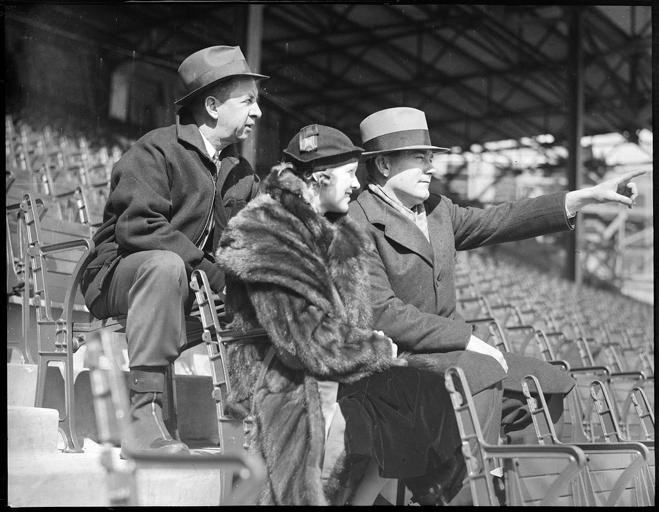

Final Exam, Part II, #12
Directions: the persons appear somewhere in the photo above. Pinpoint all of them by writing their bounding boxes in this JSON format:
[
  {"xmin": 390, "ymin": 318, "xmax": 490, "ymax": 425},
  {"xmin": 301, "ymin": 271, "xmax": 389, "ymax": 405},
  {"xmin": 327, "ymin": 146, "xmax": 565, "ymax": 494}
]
[
  {"xmin": 214, "ymin": 124, "xmax": 467, "ymax": 506},
  {"xmin": 79, "ymin": 45, "xmax": 270, "ymax": 460},
  {"xmin": 347, "ymin": 107, "xmax": 646, "ymax": 474}
]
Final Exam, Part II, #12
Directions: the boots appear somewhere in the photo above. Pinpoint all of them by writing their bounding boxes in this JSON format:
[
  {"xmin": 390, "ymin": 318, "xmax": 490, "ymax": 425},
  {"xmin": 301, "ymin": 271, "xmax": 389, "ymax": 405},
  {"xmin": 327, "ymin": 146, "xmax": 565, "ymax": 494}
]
[{"xmin": 119, "ymin": 366, "xmax": 190, "ymax": 461}]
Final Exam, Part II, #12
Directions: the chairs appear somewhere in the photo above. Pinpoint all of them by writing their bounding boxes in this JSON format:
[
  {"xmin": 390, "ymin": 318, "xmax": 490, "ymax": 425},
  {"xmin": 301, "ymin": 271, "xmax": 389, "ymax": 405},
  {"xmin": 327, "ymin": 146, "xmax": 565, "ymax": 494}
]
[{"xmin": 5, "ymin": 113, "xmax": 654, "ymax": 506}]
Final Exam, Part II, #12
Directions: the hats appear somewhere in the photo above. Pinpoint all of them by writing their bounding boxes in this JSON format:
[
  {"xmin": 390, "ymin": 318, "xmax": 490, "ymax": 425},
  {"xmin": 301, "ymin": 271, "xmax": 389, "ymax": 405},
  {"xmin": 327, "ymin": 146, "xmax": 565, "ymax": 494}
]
[
  {"xmin": 174, "ymin": 45, "xmax": 269, "ymax": 104},
  {"xmin": 361, "ymin": 106, "xmax": 451, "ymax": 154},
  {"xmin": 282, "ymin": 124, "xmax": 365, "ymax": 172}
]
